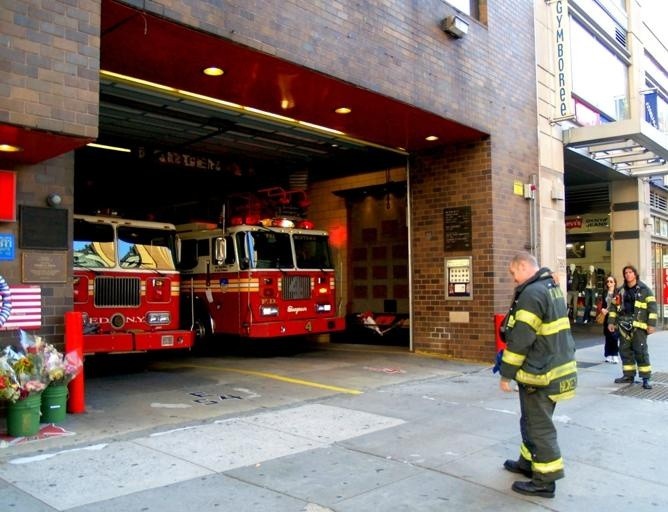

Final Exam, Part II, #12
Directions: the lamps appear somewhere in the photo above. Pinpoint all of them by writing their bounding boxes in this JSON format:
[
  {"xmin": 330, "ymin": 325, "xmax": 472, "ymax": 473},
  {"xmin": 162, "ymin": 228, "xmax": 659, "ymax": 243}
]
[{"xmin": 47, "ymin": 191, "xmax": 63, "ymax": 207}]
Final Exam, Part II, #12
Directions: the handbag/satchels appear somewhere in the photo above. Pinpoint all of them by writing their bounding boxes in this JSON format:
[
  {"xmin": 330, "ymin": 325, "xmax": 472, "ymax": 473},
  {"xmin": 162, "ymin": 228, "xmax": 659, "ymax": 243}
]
[{"xmin": 596, "ymin": 312, "xmax": 605, "ymax": 325}]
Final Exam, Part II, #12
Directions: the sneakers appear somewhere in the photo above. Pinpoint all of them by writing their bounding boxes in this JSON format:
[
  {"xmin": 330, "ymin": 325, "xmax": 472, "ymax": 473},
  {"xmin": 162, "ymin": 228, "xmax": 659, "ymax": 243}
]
[
  {"xmin": 612, "ymin": 356, "xmax": 618, "ymax": 364},
  {"xmin": 605, "ymin": 356, "xmax": 612, "ymax": 362}
]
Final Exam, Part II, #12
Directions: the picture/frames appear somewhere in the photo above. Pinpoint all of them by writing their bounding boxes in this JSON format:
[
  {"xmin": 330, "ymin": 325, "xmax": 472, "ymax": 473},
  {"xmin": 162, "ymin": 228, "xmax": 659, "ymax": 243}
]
[
  {"xmin": 18, "ymin": 202, "xmax": 69, "ymax": 251},
  {"xmin": 22, "ymin": 251, "xmax": 68, "ymax": 284},
  {"xmin": 0, "ymin": 169, "xmax": 17, "ymax": 223}
]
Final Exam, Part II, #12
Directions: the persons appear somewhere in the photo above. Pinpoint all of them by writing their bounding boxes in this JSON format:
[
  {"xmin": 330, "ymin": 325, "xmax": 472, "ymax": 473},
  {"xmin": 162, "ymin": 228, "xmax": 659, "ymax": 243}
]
[
  {"xmin": 581, "ymin": 265, "xmax": 599, "ymax": 324},
  {"xmin": 498, "ymin": 250, "xmax": 578, "ymax": 498},
  {"xmin": 567, "ymin": 264, "xmax": 579, "ymax": 324},
  {"xmin": 607, "ymin": 265, "xmax": 658, "ymax": 389},
  {"xmin": 600, "ymin": 276, "xmax": 619, "ymax": 363}
]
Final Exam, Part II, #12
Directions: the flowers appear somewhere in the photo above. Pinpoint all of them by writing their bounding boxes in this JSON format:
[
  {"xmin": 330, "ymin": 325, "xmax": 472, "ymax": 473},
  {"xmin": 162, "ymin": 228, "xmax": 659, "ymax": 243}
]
[{"xmin": 0, "ymin": 329, "xmax": 84, "ymax": 404}]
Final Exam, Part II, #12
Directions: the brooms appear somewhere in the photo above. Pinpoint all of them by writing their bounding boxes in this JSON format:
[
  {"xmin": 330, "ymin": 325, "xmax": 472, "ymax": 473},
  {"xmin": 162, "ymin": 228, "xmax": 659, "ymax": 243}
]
[{"xmin": 596, "ymin": 288, "xmax": 625, "ymax": 324}]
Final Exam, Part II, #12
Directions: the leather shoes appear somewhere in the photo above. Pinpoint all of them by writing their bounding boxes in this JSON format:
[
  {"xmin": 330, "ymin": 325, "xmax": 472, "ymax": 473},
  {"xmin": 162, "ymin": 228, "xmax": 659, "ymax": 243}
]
[
  {"xmin": 615, "ymin": 376, "xmax": 634, "ymax": 383},
  {"xmin": 503, "ymin": 460, "xmax": 532, "ymax": 478},
  {"xmin": 512, "ymin": 482, "xmax": 556, "ymax": 497},
  {"xmin": 643, "ymin": 378, "xmax": 652, "ymax": 389}
]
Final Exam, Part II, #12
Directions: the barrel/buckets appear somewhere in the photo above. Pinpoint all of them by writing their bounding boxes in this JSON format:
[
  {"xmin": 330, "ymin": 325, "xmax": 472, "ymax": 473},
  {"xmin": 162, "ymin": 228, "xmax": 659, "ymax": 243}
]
[
  {"xmin": 39, "ymin": 384, "xmax": 70, "ymax": 423},
  {"xmin": 5, "ymin": 391, "xmax": 43, "ymax": 437}
]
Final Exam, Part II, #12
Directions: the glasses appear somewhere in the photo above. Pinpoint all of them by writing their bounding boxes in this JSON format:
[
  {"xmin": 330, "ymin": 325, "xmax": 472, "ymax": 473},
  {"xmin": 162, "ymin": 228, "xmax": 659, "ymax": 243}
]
[{"xmin": 606, "ymin": 280, "xmax": 612, "ymax": 283}]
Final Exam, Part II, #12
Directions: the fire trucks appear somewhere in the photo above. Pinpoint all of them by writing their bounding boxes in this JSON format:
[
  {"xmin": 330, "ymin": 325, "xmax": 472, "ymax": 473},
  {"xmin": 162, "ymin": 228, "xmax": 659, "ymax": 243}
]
[
  {"xmin": 173, "ymin": 204, "xmax": 346, "ymax": 349},
  {"xmin": 73, "ymin": 208, "xmax": 195, "ymax": 357}
]
